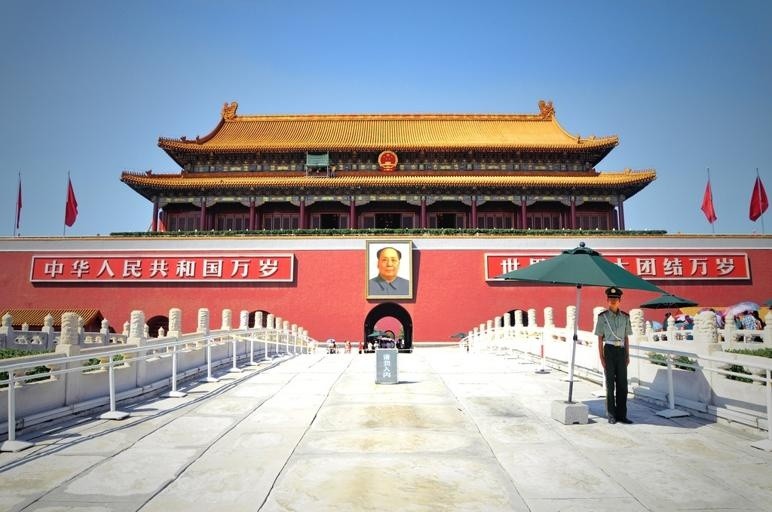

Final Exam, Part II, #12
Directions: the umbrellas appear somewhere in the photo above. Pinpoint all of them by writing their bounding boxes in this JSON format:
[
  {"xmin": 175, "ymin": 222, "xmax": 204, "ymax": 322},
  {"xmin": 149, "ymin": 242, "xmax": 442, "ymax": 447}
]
[
  {"xmin": 367, "ymin": 331, "xmax": 386, "ymax": 347},
  {"xmin": 448, "ymin": 332, "xmax": 470, "ymax": 338},
  {"xmin": 639, "ymin": 293, "xmax": 698, "ymax": 309}
]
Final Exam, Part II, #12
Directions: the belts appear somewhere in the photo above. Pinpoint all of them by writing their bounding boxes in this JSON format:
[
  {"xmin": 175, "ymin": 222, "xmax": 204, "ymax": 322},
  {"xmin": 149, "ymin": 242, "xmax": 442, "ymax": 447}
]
[{"xmin": 604, "ymin": 340, "xmax": 621, "ymax": 347}]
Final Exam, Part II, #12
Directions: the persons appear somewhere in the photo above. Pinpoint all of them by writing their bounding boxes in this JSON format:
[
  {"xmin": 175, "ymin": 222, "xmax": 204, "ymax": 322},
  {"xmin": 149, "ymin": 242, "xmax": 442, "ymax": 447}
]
[
  {"xmin": 594, "ymin": 286, "xmax": 635, "ymax": 425},
  {"xmin": 494, "ymin": 242, "xmax": 669, "ymax": 426},
  {"xmin": 323, "ymin": 338, "xmax": 404, "ymax": 354},
  {"xmin": 368, "ymin": 247, "xmax": 409, "ymax": 295},
  {"xmin": 663, "ymin": 299, "xmax": 772, "ymax": 340}
]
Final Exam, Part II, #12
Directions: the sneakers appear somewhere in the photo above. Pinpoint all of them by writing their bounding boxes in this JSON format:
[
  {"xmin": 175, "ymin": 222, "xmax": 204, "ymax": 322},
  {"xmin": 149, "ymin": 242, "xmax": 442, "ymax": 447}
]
[{"xmin": 608, "ymin": 415, "xmax": 633, "ymax": 424}]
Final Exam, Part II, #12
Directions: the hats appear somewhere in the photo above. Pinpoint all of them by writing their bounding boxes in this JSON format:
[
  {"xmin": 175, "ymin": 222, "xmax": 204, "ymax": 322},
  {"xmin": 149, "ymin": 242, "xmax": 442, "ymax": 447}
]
[{"xmin": 605, "ymin": 287, "xmax": 623, "ymax": 298}]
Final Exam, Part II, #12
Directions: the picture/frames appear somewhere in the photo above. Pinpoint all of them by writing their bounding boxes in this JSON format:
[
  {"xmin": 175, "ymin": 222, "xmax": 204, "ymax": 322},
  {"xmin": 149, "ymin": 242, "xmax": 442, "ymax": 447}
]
[{"xmin": 365, "ymin": 239, "xmax": 414, "ymax": 301}]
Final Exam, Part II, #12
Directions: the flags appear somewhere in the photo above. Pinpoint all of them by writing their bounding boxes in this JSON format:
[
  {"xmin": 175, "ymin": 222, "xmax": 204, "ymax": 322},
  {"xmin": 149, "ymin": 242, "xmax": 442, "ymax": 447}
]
[
  {"xmin": 64, "ymin": 179, "xmax": 79, "ymax": 227},
  {"xmin": 15, "ymin": 182, "xmax": 24, "ymax": 228},
  {"xmin": 750, "ymin": 176, "xmax": 769, "ymax": 222},
  {"xmin": 702, "ymin": 182, "xmax": 718, "ymax": 223}
]
